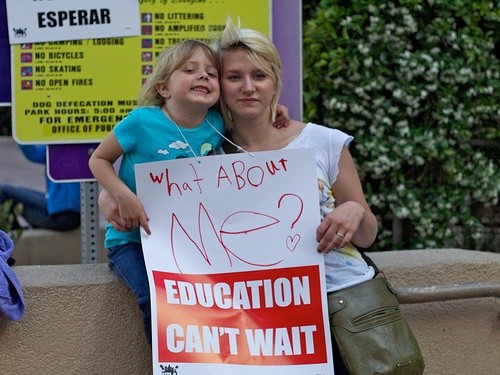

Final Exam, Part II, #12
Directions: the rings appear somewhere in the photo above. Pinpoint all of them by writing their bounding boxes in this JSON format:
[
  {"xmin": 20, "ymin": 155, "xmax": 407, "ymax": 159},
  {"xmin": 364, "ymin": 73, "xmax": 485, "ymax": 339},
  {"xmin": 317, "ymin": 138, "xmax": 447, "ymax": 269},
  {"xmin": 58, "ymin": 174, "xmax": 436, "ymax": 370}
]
[{"xmin": 337, "ymin": 232, "xmax": 345, "ymax": 239}]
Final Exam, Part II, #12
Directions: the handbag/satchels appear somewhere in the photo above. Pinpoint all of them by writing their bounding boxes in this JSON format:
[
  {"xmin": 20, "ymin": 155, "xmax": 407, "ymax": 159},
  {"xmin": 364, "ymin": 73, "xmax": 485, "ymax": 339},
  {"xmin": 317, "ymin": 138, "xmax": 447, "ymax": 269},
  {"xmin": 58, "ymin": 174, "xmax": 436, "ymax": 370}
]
[{"xmin": 328, "ymin": 272, "xmax": 424, "ymax": 374}]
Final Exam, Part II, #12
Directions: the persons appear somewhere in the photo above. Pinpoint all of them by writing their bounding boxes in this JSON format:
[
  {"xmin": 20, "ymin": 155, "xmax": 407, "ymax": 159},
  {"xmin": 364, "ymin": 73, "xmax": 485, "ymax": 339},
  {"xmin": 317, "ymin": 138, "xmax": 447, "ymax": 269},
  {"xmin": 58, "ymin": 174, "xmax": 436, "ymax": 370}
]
[
  {"xmin": 86, "ymin": 37, "xmax": 290, "ymax": 351},
  {"xmin": 96, "ymin": 16, "xmax": 423, "ymax": 374}
]
